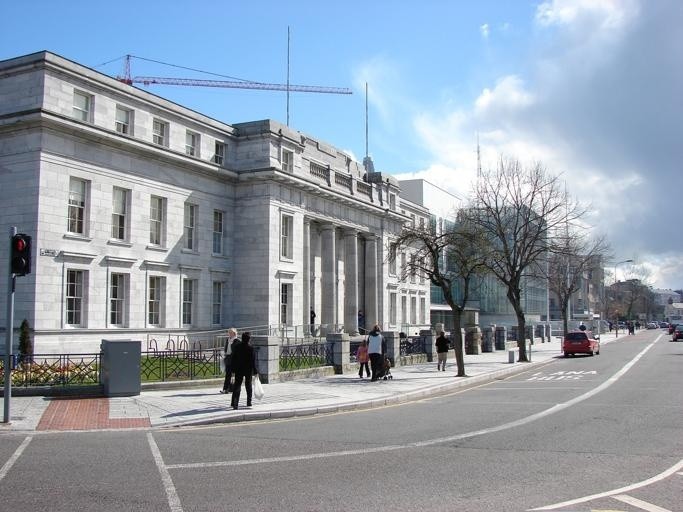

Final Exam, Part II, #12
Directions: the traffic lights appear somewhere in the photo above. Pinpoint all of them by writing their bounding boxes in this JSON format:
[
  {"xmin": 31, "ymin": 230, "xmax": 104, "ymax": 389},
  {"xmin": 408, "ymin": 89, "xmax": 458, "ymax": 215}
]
[{"xmin": 9, "ymin": 231, "xmax": 32, "ymax": 278}]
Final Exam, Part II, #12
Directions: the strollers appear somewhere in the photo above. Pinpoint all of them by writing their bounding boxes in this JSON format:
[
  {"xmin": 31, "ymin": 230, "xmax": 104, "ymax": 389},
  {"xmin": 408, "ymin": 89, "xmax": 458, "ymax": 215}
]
[{"xmin": 376, "ymin": 339, "xmax": 392, "ymax": 381}]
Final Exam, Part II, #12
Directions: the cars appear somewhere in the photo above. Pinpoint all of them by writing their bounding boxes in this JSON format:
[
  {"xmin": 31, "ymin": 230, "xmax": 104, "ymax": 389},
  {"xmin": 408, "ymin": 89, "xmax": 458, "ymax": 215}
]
[{"xmin": 606, "ymin": 315, "xmax": 682, "ymax": 342}]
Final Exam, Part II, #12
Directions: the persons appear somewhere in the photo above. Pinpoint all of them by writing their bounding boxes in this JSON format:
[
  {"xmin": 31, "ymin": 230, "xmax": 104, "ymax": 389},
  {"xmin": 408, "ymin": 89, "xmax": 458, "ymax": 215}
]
[
  {"xmin": 219, "ymin": 326, "xmax": 240, "ymax": 394},
  {"xmin": 366, "ymin": 324, "xmax": 387, "ymax": 382},
  {"xmin": 229, "ymin": 331, "xmax": 256, "ymax": 410},
  {"xmin": 357, "ymin": 310, "xmax": 364, "ymax": 332},
  {"xmin": 608, "ymin": 321, "xmax": 613, "ymax": 332},
  {"xmin": 356, "ymin": 339, "xmax": 370, "ymax": 378},
  {"xmin": 434, "ymin": 331, "xmax": 450, "ymax": 372},
  {"xmin": 628, "ymin": 321, "xmax": 641, "ymax": 335},
  {"xmin": 578, "ymin": 322, "xmax": 586, "ymax": 331},
  {"xmin": 310, "ymin": 306, "xmax": 316, "ymax": 333}
]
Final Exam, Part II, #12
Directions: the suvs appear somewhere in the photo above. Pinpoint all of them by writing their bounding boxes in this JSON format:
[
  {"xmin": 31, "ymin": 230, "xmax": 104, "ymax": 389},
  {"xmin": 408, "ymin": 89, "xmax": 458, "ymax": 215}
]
[{"xmin": 562, "ymin": 329, "xmax": 601, "ymax": 357}]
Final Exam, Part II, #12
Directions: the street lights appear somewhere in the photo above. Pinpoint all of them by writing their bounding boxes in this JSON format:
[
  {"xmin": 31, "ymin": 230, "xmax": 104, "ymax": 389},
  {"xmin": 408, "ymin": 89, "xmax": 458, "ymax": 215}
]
[{"xmin": 613, "ymin": 259, "xmax": 633, "ymax": 304}]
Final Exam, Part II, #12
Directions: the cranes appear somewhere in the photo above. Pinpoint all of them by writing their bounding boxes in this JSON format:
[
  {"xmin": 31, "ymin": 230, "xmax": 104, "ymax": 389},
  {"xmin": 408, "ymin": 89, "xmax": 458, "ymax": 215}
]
[{"xmin": 91, "ymin": 49, "xmax": 356, "ymax": 98}]
